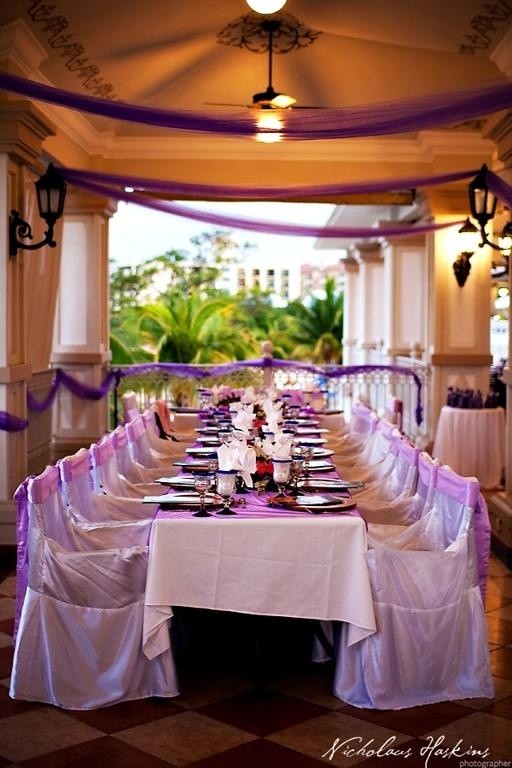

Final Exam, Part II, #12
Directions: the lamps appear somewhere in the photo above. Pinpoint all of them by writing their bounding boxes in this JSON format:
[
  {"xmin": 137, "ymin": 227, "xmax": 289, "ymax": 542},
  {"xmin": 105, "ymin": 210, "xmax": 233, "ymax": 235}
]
[
  {"xmin": 465, "ymin": 162, "xmax": 512, "ymax": 251},
  {"xmin": 452, "ymin": 216, "xmax": 482, "ymax": 287},
  {"xmin": 9, "ymin": 159, "xmax": 66, "ymax": 256}
]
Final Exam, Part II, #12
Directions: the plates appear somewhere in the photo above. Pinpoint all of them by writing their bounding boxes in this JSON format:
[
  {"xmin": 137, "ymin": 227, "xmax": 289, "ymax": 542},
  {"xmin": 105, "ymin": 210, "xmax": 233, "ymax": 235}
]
[
  {"xmin": 156, "ymin": 414, "xmax": 230, "ymax": 509},
  {"xmin": 277, "ymin": 416, "xmax": 366, "ymax": 511}
]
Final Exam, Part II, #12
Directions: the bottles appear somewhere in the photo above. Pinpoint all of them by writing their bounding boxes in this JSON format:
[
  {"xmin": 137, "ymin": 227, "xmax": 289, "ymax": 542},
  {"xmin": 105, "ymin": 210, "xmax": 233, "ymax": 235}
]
[{"xmin": 446, "ymin": 385, "xmax": 502, "ymax": 409}]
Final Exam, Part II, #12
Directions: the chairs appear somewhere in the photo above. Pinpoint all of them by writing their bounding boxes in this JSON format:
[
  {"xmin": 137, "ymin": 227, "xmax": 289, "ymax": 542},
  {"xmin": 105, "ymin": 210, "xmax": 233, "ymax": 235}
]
[{"xmin": 8, "ymin": 389, "xmax": 497, "ymax": 714}]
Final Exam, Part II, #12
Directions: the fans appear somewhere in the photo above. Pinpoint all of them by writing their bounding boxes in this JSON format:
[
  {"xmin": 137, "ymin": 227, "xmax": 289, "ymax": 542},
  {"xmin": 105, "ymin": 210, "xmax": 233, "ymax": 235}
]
[{"xmin": 200, "ymin": 8, "xmax": 332, "ymax": 114}]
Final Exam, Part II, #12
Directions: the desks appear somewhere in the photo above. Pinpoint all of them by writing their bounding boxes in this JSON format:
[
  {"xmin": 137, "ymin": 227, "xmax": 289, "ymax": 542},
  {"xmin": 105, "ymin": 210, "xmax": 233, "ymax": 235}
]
[{"xmin": 431, "ymin": 405, "xmax": 506, "ymax": 492}]
